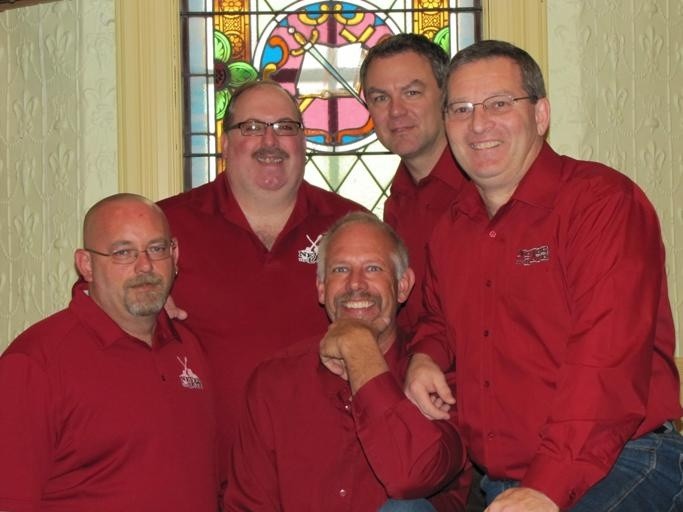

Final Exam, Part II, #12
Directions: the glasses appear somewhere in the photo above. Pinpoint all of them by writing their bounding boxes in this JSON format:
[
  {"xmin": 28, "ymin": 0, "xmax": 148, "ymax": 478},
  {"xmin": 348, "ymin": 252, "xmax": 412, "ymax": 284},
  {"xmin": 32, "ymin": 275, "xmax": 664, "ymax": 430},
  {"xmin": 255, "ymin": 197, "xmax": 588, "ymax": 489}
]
[
  {"xmin": 91, "ymin": 239, "xmax": 175, "ymax": 267},
  {"xmin": 225, "ymin": 119, "xmax": 302, "ymax": 138},
  {"xmin": 441, "ymin": 93, "xmax": 534, "ymax": 122}
]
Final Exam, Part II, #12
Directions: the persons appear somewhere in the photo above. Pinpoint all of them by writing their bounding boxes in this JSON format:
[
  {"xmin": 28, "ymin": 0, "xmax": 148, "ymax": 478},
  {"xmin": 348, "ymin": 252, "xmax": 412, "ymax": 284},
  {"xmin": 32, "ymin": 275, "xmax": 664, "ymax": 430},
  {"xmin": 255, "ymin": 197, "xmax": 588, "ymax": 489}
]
[
  {"xmin": 156, "ymin": 80, "xmax": 378, "ymax": 480},
  {"xmin": 0, "ymin": 193, "xmax": 219, "ymax": 512},
  {"xmin": 357, "ymin": 33, "xmax": 472, "ymax": 367},
  {"xmin": 399, "ymin": 38, "xmax": 682, "ymax": 512},
  {"xmin": 220, "ymin": 210, "xmax": 474, "ymax": 511}
]
